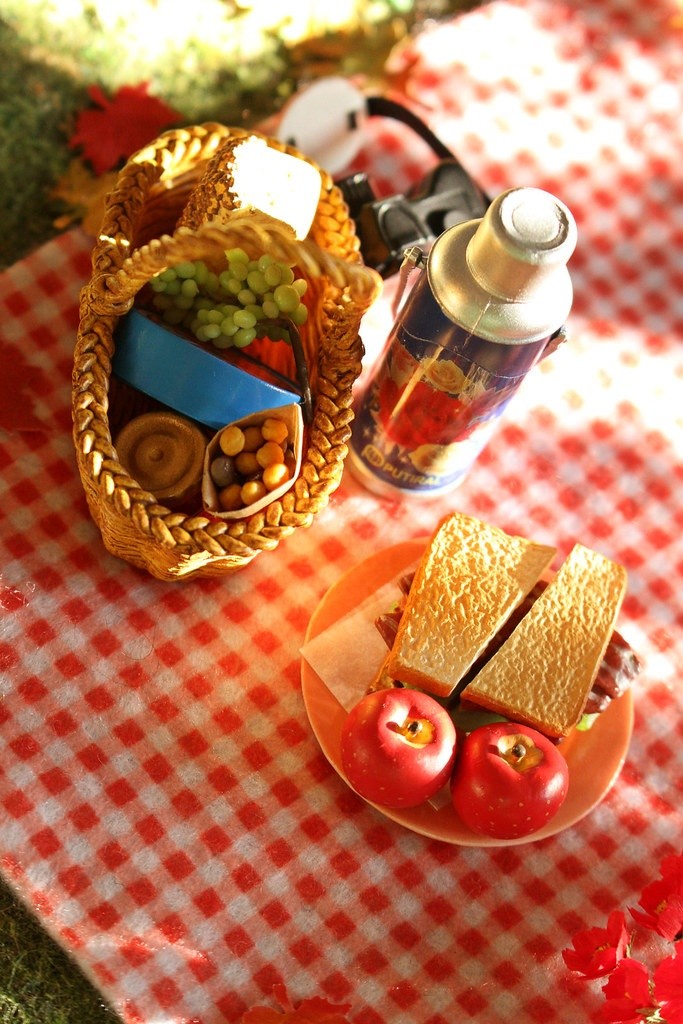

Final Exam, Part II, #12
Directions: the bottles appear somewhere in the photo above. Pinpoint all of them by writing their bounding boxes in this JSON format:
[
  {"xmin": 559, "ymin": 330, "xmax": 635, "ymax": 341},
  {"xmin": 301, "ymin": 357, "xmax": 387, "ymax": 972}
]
[{"xmin": 346, "ymin": 185, "xmax": 577, "ymax": 504}]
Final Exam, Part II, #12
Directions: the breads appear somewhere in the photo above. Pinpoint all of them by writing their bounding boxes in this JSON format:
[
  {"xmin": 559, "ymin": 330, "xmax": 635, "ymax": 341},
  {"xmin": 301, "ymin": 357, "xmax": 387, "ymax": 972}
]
[
  {"xmin": 176, "ymin": 136, "xmax": 323, "ymax": 242},
  {"xmin": 387, "ymin": 510, "xmax": 557, "ymax": 695},
  {"xmin": 460, "ymin": 544, "xmax": 627, "ymax": 738}
]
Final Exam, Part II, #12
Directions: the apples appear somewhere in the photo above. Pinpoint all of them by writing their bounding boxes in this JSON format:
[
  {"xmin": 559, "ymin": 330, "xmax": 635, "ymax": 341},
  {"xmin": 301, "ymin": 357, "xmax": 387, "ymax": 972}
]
[
  {"xmin": 338, "ymin": 689, "xmax": 460, "ymax": 809},
  {"xmin": 448, "ymin": 721, "xmax": 568, "ymax": 839}
]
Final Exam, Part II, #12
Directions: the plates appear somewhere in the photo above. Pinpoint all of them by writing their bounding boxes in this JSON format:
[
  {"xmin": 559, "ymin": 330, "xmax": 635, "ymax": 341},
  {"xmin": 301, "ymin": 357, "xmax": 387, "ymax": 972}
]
[{"xmin": 300, "ymin": 534, "xmax": 635, "ymax": 847}]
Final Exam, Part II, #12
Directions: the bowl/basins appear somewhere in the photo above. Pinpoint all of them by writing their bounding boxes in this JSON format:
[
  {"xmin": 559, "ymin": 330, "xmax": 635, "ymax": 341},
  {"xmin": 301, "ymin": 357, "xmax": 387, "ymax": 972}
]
[{"xmin": 114, "ymin": 305, "xmax": 305, "ymax": 433}]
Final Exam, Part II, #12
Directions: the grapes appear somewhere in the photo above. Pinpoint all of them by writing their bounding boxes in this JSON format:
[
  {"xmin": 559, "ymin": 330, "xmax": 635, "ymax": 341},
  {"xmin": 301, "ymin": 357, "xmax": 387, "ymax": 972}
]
[{"xmin": 146, "ymin": 247, "xmax": 308, "ymax": 348}]
